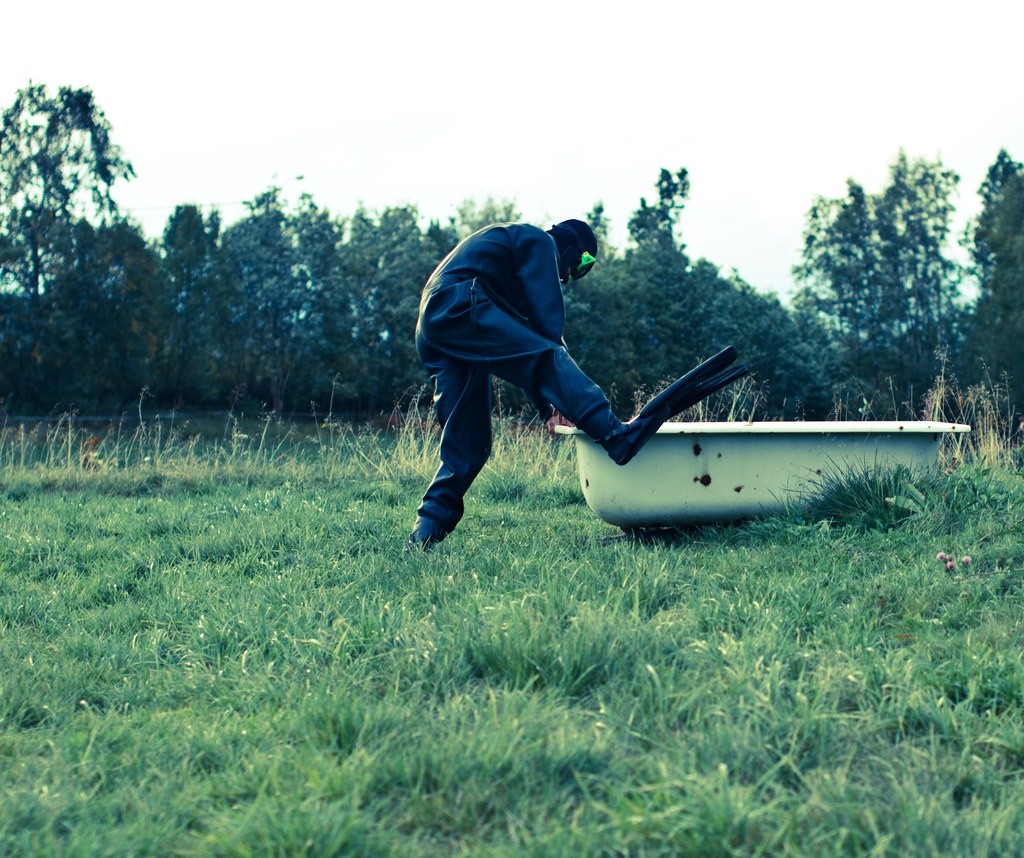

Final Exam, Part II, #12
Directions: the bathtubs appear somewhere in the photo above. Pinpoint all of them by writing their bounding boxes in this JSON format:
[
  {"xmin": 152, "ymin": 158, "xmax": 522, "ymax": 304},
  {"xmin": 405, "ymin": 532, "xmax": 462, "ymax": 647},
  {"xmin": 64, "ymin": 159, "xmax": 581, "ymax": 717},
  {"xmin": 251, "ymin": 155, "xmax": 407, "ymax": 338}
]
[{"xmin": 552, "ymin": 421, "xmax": 971, "ymax": 532}]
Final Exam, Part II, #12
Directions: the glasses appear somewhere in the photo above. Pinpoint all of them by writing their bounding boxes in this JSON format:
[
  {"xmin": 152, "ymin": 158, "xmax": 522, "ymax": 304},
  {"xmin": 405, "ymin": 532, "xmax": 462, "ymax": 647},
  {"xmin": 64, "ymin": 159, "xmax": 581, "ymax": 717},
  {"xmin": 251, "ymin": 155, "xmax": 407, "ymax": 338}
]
[{"xmin": 574, "ymin": 251, "xmax": 595, "ymax": 282}]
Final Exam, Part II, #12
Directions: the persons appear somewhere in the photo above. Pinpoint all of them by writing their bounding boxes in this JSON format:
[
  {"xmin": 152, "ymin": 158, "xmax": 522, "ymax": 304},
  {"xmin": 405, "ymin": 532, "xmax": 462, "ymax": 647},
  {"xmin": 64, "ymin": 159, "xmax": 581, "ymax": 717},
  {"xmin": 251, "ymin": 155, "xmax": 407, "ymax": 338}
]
[{"xmin": 404, "ymin": 219, "xmax": 751, "ymax": 556}]
